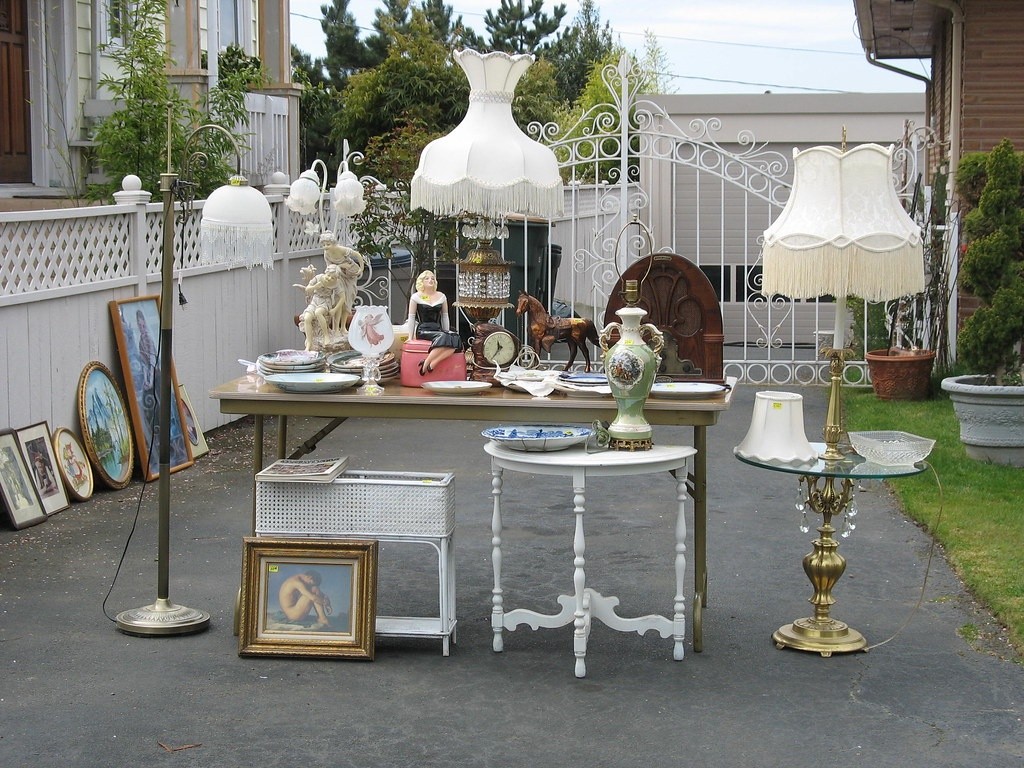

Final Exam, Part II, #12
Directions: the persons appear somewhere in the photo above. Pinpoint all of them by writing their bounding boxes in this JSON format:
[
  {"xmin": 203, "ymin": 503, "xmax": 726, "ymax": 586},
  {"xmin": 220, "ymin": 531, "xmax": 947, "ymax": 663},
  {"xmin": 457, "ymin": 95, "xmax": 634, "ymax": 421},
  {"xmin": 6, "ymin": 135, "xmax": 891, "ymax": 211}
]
[
  {"xmin": 404, "ymin": 271, "xmax": 461, "ymax": 375},
  {"xmin": 320, "ymin": 232, "xmax": 364, "ymax": 334},
  {"xmin": 303, "ymin": 264, "xmax": 346, "ymax": 352}
]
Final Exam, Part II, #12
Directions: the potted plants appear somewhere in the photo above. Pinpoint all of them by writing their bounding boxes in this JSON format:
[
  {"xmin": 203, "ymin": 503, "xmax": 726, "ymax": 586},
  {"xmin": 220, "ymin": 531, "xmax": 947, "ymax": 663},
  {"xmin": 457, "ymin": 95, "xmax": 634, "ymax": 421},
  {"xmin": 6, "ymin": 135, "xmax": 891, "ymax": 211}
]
[
  {"xmin": 941, "ymin": 139, "xmax": 1024, "ymax": 466},
  {"xmin": 866, "ymin": 160, "xmax": 947, "ymax": 399}
]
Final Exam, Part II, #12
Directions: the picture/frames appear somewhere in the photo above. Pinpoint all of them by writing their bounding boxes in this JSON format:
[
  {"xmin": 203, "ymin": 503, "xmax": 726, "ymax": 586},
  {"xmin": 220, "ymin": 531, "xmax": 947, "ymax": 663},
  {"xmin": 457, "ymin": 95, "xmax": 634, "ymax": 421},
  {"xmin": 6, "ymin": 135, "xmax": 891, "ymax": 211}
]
[
  {"xmin": 17, "ymin": 421, "xmax": 70, "ymax": 517},
  {"xmin": 0, "ymin": 427, "xmax": 48, "ymax": 531},
  {"xmin": 238, "ymin": 537, "xmax": 379, "ymax": 662},
  {"xmin": 108, "ymin": 294, "xmax": 195, "ymax": 482},
  {"xmin": 178, "ymin": 384, "xmax": 209, "ymax": 459}
]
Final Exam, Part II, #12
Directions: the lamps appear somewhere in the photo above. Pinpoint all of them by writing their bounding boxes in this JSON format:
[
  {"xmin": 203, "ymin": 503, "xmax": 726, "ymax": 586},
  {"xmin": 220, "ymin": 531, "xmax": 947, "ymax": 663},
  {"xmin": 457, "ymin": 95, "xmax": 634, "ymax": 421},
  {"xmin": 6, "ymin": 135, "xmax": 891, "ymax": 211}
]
[
  {"xmin": 762, "ymin": 127, "xmax": 924, "ymax": 460},
  {"xmin": 411, "ymin": 48, "xmax": 564, "ymax": 367},
  {"xmin": 283, "ymin": 139, "xmax": 365, "ymax": 356},
  {"xmin": 101, "ymin": 101, "xmax": 277, "ymax": 638}
]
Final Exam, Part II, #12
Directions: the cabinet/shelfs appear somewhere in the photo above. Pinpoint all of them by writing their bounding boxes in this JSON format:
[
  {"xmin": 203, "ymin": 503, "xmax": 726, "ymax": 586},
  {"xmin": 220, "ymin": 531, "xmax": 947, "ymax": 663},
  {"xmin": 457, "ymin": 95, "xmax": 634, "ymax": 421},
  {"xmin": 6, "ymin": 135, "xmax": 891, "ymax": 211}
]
[{"xmin": 258, "ymin": 472, "xmax": 459, "ymax": 657}]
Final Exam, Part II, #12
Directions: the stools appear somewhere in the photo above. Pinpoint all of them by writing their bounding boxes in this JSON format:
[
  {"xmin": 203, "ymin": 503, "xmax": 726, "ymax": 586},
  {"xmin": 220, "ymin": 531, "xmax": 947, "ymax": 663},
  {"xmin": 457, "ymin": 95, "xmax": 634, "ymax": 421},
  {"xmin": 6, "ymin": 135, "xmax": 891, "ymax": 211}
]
[{"xmin": 402, "ymin": 341, "xmax": 469, "ymax": 385}]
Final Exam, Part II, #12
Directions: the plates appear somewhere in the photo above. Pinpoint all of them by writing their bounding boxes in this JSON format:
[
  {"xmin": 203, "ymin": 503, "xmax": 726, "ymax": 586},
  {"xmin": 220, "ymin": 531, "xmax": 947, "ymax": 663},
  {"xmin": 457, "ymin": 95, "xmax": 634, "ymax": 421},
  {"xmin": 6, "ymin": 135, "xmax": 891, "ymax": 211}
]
[
  {"xmin": 257, "ymin": 349, "xmax": 398, "ymax": 393},
  {"xmin": 497, "ymin": 371, "xmax": 612, "ymax": 397},
  {"xmin": 482, "ymin": 427, "xmax": 595, "ymax": 452},
  {"xmin": 650, "ymin": 383, "xmax": 724, "ymax": 396},
  {"xmin": 421, "ymin": 380, "xmax": 492, "ymax": 395}
]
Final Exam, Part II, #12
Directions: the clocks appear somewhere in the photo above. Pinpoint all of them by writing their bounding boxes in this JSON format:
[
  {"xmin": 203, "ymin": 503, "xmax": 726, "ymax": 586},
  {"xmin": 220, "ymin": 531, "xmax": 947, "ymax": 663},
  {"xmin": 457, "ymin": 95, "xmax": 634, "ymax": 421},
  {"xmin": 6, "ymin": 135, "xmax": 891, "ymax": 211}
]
[{"xmin": 468, "ymin": 322, "xmax": 520, "ymax": 385}]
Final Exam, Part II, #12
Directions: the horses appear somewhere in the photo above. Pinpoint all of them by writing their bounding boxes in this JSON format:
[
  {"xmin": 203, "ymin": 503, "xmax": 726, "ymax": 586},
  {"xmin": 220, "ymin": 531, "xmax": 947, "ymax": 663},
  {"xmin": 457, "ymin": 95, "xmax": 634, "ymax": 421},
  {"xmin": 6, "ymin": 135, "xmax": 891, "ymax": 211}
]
[{"xmin": 515, "ymin": 289, "xmax": 601, "ymax": 372}]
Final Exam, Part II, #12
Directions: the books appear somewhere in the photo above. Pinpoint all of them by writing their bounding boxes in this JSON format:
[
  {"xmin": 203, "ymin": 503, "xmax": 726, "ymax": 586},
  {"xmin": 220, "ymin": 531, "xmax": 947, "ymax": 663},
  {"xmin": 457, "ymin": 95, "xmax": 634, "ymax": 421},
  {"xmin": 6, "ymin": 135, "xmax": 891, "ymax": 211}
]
[{"xmin": 255, "ymin": 457, "xmax": 349, "ymax": 484}]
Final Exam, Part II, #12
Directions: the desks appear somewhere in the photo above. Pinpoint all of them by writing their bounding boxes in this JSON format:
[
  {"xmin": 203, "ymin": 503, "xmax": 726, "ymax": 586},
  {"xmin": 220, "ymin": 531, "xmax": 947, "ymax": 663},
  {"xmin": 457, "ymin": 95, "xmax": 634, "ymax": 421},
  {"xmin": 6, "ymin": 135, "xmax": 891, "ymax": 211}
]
[
  {"xmin": 211, "ymin": 373, "xmax": 738, "ymax": 655},
  {"xmin": 485, "ymin": 441, "xmax": 698, "ymax": 678},
  {"xmin": 736, "ymin": 443, "xmax": 927, "ymax": 658}
]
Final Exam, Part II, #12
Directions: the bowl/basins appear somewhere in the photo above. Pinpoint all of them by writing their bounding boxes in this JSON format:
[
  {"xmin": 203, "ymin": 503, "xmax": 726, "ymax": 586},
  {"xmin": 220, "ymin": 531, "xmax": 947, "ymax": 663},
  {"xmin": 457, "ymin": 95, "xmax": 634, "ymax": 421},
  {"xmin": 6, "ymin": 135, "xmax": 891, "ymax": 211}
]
[{"xmin": 848, "ymin": 429, "xmax": 936, "ymax": 466}]
